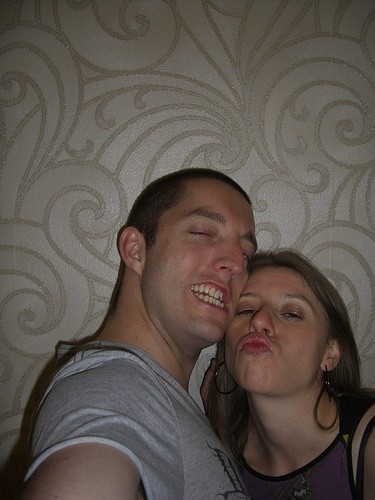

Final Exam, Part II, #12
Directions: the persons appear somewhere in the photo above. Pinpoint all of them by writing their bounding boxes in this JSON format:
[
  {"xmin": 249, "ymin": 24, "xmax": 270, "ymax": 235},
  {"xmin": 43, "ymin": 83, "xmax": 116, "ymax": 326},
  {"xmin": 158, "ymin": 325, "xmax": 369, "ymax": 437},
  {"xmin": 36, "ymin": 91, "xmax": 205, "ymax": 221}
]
[
  {"xmin": 206, "ymin": 250, "xmax": 375, "ymax": 500},
  {"xmin": 21, "ymin": 169, "xmax": 257, "ymax": 500}
]
